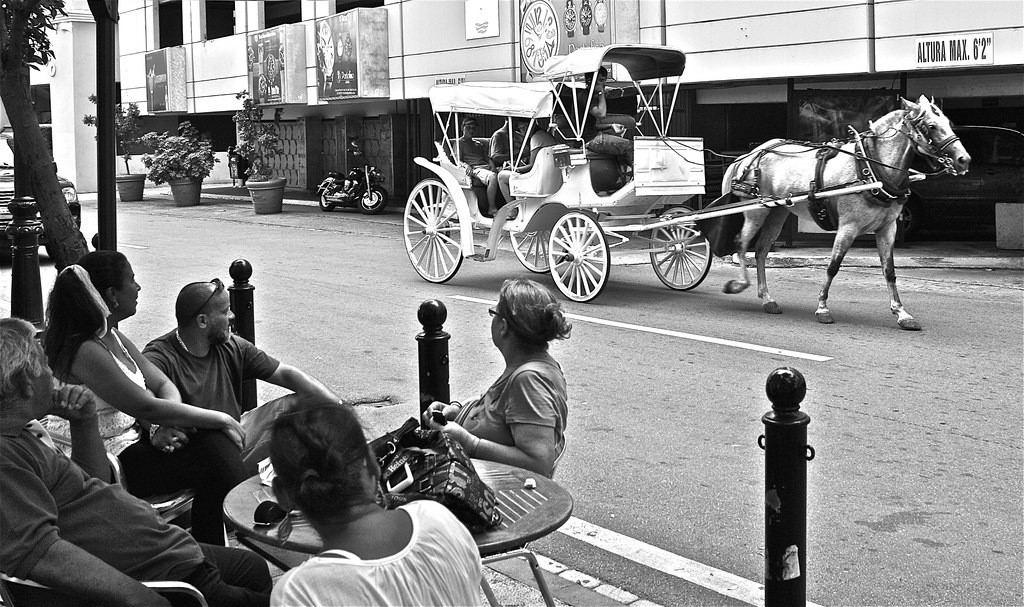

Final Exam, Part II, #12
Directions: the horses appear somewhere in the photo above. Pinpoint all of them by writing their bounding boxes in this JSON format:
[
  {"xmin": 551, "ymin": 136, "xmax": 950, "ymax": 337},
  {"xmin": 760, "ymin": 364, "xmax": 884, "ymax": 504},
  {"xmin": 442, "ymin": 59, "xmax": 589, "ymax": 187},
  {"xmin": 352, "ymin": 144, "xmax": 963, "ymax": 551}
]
[{"xmin": 721, "ymin": 93, "xmax": 972, "ymax": 329}]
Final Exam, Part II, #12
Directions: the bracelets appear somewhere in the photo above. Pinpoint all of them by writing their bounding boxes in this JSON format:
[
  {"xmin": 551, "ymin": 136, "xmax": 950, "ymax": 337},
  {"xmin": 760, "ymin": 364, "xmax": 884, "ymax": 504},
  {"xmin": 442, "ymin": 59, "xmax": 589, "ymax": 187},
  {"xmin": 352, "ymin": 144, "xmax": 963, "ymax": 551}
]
[
  {"xmin": 470, "ymin": 436, "xmax": 480, "ymax": 457},
  {"xmin": 448, "ymin": 400, "xmax": 462, "ymax": 409},
  {"xmin": 336, "ymin": 399, "xmax": 347, "ymax": 406},
  {"xmin": 149, "ymin": 423, "xmax": 159, "ymax": 442}
]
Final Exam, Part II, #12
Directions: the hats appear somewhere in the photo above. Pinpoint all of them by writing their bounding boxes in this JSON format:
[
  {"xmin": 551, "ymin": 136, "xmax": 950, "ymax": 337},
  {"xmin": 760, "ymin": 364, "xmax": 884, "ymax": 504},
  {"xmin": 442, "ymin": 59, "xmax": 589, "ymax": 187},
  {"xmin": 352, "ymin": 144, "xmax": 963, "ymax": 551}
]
[{"xmin": 462, "ymin": 117, "xmax": 480, "ymax": 131}]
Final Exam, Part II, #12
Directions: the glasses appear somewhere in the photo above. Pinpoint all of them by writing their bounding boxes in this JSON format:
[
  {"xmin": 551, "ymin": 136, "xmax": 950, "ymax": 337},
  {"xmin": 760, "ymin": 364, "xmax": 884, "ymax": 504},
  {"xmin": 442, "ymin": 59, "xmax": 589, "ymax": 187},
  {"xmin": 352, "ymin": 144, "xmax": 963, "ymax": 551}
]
[
  {"xmin": 488, "ymin": 307, "xmax": 505, "ymax": 319},
  {"xmin": 188, "ymin": 278, "xmax": 225, "ymax": 318},
  {"xmin": 255, "ymin": 501, "xmax": 283, "ymax": 526}
]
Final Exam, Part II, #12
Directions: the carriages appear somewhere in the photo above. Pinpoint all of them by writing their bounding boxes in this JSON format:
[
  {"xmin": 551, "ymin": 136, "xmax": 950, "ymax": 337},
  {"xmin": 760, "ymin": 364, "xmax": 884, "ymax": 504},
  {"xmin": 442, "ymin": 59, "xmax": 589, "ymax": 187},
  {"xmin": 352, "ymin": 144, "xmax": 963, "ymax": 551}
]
[{"xmin": 403, "ymin": 43, "xmax": 973, "ymax": 329}]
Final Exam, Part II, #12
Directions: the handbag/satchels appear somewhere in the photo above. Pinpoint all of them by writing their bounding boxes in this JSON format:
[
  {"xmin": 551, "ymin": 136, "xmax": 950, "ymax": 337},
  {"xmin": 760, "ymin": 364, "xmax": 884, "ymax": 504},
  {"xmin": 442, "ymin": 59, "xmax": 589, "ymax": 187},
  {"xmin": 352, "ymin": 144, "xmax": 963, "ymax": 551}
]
[{"xmin": 369, "ymin": 417, "xmax": 503, "ymax": 534}]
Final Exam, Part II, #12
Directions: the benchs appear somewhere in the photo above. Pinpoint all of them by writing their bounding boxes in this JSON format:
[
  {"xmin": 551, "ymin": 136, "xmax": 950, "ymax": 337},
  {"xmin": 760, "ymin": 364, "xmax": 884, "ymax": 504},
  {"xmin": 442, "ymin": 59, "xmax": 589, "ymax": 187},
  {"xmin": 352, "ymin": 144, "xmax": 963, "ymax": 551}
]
[
  {"xmin": 552, "ymin": 111, "xmax": 644, "ymax": 159},
  {"xmin": 431, "ymin": 137, "xmax": 502, "ymax": 187},
  {"xmin": 510, "ymin": 144, "xmax": 566, "ymax": 196}
]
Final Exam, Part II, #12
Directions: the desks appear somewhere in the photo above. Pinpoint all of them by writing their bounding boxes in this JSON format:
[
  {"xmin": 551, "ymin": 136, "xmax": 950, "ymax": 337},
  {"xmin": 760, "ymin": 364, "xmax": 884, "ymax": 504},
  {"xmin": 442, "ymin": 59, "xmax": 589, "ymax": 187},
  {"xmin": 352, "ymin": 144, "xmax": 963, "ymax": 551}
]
[{"xmin": 222, "ymin": 444, "xmax": 574, "ymax": 607}]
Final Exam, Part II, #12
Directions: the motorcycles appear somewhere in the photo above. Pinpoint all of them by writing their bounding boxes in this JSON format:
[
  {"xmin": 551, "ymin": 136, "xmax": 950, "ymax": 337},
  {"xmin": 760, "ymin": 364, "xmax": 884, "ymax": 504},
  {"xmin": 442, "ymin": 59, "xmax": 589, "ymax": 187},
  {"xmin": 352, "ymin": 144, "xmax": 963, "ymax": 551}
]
[{"xmin": 315, "ymin": 141, "xmax": 389, "ymax": 215}]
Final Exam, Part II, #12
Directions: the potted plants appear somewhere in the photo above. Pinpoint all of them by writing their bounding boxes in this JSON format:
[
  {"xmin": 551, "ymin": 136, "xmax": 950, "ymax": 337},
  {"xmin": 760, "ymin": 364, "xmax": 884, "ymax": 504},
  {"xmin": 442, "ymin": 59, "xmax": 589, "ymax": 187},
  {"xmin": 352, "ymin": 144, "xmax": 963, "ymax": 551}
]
[
  {"xmin": 135, "ymin": 120, "xmax": 221, "ymax": 206},
  {"xmin": 232, "ymin": 90, "xmax": 288, "ymax": 213},
  {"xmin": 81, "ymin": 93, "xmax": 148, "ymax": 202}
]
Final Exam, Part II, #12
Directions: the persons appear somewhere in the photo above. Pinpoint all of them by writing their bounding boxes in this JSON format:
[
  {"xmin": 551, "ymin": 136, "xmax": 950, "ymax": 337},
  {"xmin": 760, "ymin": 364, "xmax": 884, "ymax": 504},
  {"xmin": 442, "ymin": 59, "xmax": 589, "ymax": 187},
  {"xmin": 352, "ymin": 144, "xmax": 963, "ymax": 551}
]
[
  {"xmin": 570, "ymin": 66, "xmax": 633, "ymax": 170},
  {"xmin": 142, "ymin": 277, "xmax": 348, "ymax": 467},
  {"xmin": 489, "ymin": 113, "xmax": 558, "ymax": 220},
  {"xmin": 0, "ymin": 318, "xmax": 272, "ymax": 607},
  {"xmin": 453, "ymin": 117, "xmax": 498, "ymax": 217},
  {"xmin": 39, "ymin": 251, "xmax": 244, "ymax": 553},
  {"xmin": 267, "ymin": 396, "xmax": 483, "ymax": 607},
  {"xmin": 423, "ymin": 277, "xmax": 573, "ymax": 475}
]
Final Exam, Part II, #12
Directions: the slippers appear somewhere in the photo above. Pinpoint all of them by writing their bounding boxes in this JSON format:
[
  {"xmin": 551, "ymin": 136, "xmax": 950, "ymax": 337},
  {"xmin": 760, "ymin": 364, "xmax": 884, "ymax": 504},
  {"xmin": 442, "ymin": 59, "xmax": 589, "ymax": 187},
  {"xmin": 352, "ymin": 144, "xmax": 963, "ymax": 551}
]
[
  {"xmin": 506, "ymin": 215, "xmax": 516, "ymax": 220},
  {"xmin": 488, "ymin": 210, "xmax": 498, "ymax": 217}
]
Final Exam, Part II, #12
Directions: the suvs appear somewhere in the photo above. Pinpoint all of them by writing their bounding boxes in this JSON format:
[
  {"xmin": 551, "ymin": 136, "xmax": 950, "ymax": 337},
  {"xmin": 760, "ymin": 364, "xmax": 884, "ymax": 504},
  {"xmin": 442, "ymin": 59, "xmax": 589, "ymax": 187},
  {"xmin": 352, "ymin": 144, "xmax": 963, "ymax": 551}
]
[
  {"xmin": 0, "ymin": 131, "xmax": 81, "ymax": 264},
  {"xmin": 899, "ymin": 125, "xmax": 1024, "ymax": 242}
]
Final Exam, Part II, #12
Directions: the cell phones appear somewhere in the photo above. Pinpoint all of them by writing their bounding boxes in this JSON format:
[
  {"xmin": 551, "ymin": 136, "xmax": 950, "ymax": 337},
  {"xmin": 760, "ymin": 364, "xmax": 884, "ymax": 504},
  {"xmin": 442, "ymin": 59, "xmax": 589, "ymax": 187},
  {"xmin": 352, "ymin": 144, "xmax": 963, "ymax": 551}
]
[
  {"xmin": 432, "ymin": 410, "xmax": 448, "ymax": 426},
  {"xmin": 386, "ymin": 462, "xmax": 414, "ymax": 494}
]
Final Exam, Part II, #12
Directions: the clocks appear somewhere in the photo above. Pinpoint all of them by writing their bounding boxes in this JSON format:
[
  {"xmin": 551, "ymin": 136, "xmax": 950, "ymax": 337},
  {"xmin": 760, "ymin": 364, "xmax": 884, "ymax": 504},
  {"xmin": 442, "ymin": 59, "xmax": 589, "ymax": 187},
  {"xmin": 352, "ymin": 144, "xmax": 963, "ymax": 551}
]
[{"xmin": 517, "ymin": 0, "xmax": 608, "ymax": 75}]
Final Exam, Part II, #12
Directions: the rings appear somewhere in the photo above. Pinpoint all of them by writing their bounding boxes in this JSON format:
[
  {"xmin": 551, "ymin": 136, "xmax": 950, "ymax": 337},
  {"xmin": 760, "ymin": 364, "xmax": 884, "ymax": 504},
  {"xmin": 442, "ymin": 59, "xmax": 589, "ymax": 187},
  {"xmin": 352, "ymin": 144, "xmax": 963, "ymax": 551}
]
[{"xmin": 162, "ymin": 446, "xmax": 169, "ymax": 452}]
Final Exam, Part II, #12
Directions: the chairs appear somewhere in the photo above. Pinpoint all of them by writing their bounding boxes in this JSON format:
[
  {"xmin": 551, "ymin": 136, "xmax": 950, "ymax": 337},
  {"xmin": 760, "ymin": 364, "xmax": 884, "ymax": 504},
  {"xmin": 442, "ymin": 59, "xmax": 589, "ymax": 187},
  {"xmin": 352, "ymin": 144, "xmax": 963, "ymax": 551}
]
[{"xmin": 0, "ymin": 332, "xmax": 567, "ymax": 607}]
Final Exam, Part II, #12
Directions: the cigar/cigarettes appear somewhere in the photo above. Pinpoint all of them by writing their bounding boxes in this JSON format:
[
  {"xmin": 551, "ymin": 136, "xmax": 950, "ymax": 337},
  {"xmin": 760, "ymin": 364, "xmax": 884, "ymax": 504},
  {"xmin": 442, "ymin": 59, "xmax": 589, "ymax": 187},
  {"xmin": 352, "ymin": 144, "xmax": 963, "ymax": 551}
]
[{"xmin": 172, "ymin": 437, "xmax": 179, "ymax": 441}]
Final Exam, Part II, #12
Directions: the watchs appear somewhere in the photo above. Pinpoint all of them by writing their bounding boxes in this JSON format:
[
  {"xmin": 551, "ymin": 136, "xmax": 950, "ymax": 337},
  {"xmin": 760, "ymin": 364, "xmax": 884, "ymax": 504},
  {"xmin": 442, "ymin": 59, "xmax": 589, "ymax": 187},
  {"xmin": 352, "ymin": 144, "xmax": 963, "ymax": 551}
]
[
  {"xmin": 317, "ymin": 20, "xmax": 352, "ymax": 91},
  {"xmin": 564, "ymin": 0, "xmax": 608, "ymax": 37},
  {"xmin": 598, "ymin": 91, "xmax": 604, "ymax": 95},
  {"xmin": 520, "ymin": 0, "xmax": 561, "ymax": 82},
  {"xmin": 247, "ymin": 28, "xmax": 286, "ymax": 104}
]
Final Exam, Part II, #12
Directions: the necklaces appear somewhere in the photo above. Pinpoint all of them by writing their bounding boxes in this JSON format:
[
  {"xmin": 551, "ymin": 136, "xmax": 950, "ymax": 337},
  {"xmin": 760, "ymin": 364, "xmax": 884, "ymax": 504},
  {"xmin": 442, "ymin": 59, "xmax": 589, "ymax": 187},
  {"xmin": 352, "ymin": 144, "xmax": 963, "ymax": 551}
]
[{"xmin": 175, "ymin": 329, "xmax": 189, "ymax": 352}]
